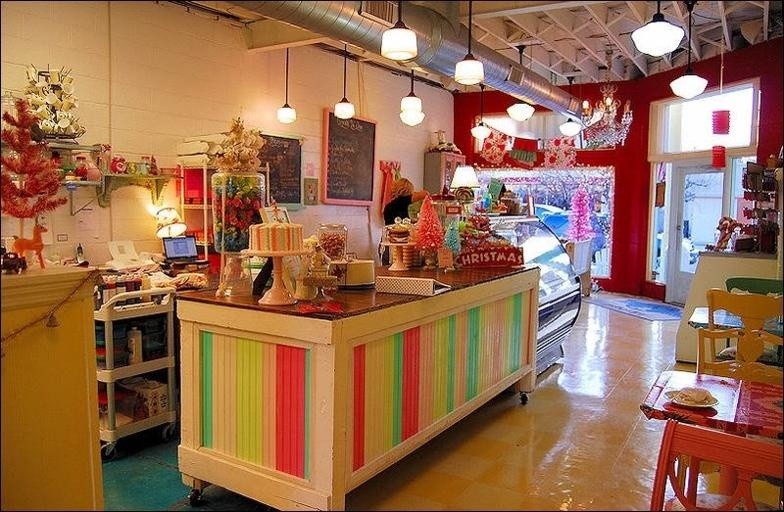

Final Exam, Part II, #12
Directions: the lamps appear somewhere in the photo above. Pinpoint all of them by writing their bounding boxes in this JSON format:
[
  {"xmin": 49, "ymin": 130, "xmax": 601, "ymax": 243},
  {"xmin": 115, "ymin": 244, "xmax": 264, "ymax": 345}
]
[
  {"xmin": 506, "ymin": 44, "xmax": 535, "ymax": 123},
  {"xmin": 669, "ymin": 1, "xmax": 709, "ymax": 100},
  {"xmin": 334, "ymin": 43, "xmax": 355, "ymax": 121},
  {"xmin": 630, "ymin": 1, "xmax": 685, "ymax": 58},
  {"xmin": 470, "ymin": 83, "xmax": 492, "ymax": 140},
  {"xmin": 578, "ymin": 50, "xmax": 634, "ymax": 148},
  {"xmin": 400, "ymin": 69, "xmax": 425, "ymax": 127},
  {"xmin": 558, "ymin": 76, "xmax": 582, "ymax": 138},
  {"xmin": 455, "ymin": 2, "xmax": 485, "ymax": 87},
  {"xmin": 280, "ymin": 47, "xmax": 296, "ymax": 125},
  {"xmin": 155, "ymin": 208, "xmax": 188, "ymax": 239},
  {"xmin": 380, "ymin": 2, "xmax": 417, "ymax": 62},
  {"xmin": 450, "ymin": 165, "xmax": 480, "ymax": 202}
]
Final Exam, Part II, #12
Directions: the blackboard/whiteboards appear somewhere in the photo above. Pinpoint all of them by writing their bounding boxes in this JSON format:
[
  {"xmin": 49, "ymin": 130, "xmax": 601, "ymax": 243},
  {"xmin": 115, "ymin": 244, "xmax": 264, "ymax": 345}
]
[
  {"xmin": 319, "ymin": 106, "xmax": 377, "ymax": 206},
  {"xmin": 256, "ymin": 129, "xmax": 305, "ymax": 211}
]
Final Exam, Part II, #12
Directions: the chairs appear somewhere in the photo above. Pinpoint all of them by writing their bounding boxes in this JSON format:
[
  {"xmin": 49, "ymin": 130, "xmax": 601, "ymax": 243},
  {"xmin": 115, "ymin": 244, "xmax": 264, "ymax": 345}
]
[
  {"xmin": 565, "ymin": 238, "xmax": 592, "ymax": 296},
  {"xmin": 648, "ymin": 418, "xmax": 783, "ymax": 511},
  {"xmin": 697, "ymin": 326, "xmax": 783, "ymax": 386},
  {"xmin": 707, "ymin": 288, "xmax": 784, "ymax": 362},
  {"xmin": 724, "ymin": 278, "xmax": 784, "ymax": 294}
]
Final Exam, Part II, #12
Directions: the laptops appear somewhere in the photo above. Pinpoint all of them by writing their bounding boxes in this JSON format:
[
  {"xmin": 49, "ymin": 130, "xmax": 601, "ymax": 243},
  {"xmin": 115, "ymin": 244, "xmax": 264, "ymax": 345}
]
[{"xmin": 162, "ymin": 235, "xmax": 209, "ymax": 266}]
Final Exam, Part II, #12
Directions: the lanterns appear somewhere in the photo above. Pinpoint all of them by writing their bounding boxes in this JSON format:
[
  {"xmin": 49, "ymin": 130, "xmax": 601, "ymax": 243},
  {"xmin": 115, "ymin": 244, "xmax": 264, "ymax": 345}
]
[
  {"xmin": 711, "ymin": 144, "xmax": 727, "ymax": 171},
  {"xmin": 710, "ymin": 110, "xmax": 731, "ymax": 136}
]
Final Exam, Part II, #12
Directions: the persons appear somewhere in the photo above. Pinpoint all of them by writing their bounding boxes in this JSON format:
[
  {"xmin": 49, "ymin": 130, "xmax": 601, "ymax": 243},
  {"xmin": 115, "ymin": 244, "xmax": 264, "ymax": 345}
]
[{"xmin": 378, "ymin": 178, "xmax": 430, "ymax": 266}]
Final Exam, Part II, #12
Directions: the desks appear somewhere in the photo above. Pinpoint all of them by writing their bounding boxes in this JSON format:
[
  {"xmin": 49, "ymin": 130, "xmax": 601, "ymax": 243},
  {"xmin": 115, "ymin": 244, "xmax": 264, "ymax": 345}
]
[
  {"xmin": 175, "ymin": 263, "xmax": 542, "ymax": 512},
  {"xmin": 676, "ymin": 252, "xmax": 777, "ymax": 363},
  {"xmin": 0, "ymin": 264, "xmax": 105, "ymax": 512}
]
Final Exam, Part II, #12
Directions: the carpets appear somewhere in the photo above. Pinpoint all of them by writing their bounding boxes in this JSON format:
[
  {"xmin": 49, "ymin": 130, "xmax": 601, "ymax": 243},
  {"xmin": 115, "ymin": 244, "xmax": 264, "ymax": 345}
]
[{"xmin": 582, "ymin": 297, "xmax": 682, "ymax": 321}]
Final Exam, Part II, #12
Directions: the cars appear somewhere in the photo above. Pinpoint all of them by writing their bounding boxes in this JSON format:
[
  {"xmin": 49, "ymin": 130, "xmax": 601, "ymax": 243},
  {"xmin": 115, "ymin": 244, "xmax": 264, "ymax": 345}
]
[
  {"xmin": 540, "ymin": 207, "xmax": 610, "ymax": 263},
  {"xmin": 656, "ymin": 232, "xmax": 700, "ymax": 266},
  {"xmin": 521, "ymin": 203, "xmax": 562, "ymax": 220}
]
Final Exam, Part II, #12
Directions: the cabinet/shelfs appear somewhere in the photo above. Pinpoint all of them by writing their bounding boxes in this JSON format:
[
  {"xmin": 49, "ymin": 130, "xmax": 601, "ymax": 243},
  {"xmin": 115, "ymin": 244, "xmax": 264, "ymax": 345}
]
[
  {"xmin": 93, "ymin": 306, "xmax": 176, "ymax": 443},
  {"xmin": 12, "ymin": 141, "xmax": 103, "ymax": 184},
  {"xmin": 180, "ymin": 163, "xmax": 270, "ymax": 247},
  {"xmin": 424, "ymin": 152, "xmax": 465, "ymax": 194}
]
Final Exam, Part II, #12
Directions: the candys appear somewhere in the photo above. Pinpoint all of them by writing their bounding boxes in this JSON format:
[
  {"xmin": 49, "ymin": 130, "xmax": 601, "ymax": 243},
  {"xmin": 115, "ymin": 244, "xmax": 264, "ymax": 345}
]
[
  {"xmin": 320, "ymin": 234, "xmax": 344, "ymax": 260},
  {"xmin": 212, "ymin": 175, "xmax": 261, "ymax": 252},
  {"xmin": 454, "ymin": 187, "xmax": 475, "ymax": 221},
  {"xmin": 474, "ymin": 196, "xmax": 490, "ymax": 219}
]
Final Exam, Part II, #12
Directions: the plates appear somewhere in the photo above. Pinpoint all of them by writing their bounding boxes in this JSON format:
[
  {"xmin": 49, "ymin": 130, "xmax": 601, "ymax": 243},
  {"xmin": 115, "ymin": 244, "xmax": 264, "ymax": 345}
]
[{"xmin": 663, "ymin": 390, "xmax": 721, "ymax": 408}]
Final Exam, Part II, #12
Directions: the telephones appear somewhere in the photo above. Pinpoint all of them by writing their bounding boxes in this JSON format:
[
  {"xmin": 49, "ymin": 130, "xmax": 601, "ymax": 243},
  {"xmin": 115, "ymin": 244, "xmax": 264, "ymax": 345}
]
[{"xmin": 73, "ymin": 242, "xmax": 89, "ymax": 268}]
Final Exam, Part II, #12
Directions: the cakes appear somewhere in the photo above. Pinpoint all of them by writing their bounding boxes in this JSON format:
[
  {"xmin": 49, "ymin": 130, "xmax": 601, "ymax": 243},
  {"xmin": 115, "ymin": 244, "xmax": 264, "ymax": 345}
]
[
  {"xmin": 500, "ymin": 192, "xmax": 522, "ymax": 213},
  {"xmin": 248, "ymin": 196, "xmax": 307, "ymax": 251},
  {"xmin": 328, "ymin": 259, "xmax": 374, "ymax": 285},
  {"xmin": 382, "ymin": 224, "xmax": 410, "ymax": 243}
]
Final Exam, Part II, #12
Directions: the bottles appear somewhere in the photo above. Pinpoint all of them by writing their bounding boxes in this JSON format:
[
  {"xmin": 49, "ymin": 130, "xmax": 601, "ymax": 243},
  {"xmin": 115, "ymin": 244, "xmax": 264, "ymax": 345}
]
[
  {"xmin": 111, "ymin": 157, "xmax": 129, "ymax": 174},
  {"xmin": 140, "ymin": 155, "xmax": 152, "ymax": 174},
  {"xmin": 65, "ymin": 171, "xmax": 75, "ymax": 181},
  {"xmin": 318, "ymin": 223, "xmax": 347, "ymax": 262},
  {"xmin": 94, "ymin": 271, "xmax": 154, "ymax": 307},
  {"xmin": 127, "ymin": 326, "xmax": 144, "ymax": 364},
  {"xmin": 72, "ymin": 155, "xmax": 87, "ymax": 180}
]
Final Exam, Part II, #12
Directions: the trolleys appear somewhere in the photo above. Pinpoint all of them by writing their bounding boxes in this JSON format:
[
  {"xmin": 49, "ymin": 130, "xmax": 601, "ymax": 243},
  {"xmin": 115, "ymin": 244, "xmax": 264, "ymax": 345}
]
[{"xmin": 93, "ymin": 287, "xmax": 177, "ymax": 462}]
